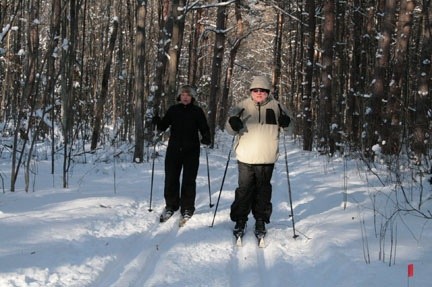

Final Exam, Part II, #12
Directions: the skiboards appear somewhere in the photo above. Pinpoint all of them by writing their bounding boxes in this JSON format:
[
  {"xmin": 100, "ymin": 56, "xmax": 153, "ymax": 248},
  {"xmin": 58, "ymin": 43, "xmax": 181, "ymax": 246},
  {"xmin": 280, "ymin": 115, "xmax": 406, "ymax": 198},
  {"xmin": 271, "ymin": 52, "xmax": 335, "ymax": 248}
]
[
  {"xmin": 159, "ymin": 204, "xmax": 194, "ymax": 229},
  {"xmin": 233, "ymin": 220, "xmax": 266, "ymax": 248}
]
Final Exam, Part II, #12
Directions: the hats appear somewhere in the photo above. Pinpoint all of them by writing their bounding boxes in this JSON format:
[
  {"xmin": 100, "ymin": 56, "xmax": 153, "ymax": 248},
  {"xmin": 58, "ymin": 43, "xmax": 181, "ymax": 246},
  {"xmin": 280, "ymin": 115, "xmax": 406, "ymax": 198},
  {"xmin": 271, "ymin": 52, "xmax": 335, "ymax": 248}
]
[{"xmin": 175, "ymin": 85, "xmax": 198, "ymax": 101}]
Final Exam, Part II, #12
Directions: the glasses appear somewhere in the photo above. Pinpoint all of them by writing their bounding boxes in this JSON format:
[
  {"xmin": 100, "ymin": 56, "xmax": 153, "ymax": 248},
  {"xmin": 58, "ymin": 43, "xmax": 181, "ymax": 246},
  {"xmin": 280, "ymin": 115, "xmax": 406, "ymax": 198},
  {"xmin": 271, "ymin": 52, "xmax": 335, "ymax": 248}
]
[{"xmin": 253, "ymin": 89, "xmax": 267, "ymax": 92}]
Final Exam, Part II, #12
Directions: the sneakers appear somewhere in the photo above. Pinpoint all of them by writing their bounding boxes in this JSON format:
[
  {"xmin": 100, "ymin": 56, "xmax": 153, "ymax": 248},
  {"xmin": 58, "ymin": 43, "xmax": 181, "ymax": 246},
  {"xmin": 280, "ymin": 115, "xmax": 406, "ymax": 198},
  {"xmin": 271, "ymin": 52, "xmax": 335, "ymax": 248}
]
[
  {"xmin": 182, "ymin": 210, "xmax": 192, "ymax": 220},
  {"xmin": 165, "ymin": 207, "xmax": 173, "ymax": 218},
  {"xmin": 254, "ymin": 220, "xmax": 266, "ymax": 238},
  {"xmin": 233, "ymin": 222, "xmax": 247, "ymax": 238}
]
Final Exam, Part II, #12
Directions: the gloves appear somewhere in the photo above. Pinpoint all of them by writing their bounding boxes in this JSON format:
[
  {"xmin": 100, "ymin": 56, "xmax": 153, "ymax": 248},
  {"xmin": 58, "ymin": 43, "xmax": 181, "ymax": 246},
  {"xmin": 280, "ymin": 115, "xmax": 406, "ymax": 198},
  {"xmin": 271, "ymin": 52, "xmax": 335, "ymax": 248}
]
[
  {"xmin": 151, "ymin": 115, "xmax": 160, "ymax": 125},
  {"xmin": 229, "ymin": 116, "xmax": 244, "ymax": 132},
  {"xmin": 277, "ymin": 115, "xmax": 291, "ymax": 128},
  {"xmin": 201, "ymin": 135, "xmax": 211, "ymax": 145}
]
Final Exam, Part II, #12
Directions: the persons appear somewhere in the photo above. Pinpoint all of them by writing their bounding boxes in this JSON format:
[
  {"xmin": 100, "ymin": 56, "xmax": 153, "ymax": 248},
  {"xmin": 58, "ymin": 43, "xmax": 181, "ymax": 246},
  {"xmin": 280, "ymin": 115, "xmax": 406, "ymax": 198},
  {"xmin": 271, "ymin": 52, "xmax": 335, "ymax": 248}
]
[
  {"xmin": 225, "ymin": 76, "xmax": 295, "ymax": 236},
  {"xmin": 151, "ymin": 85, "xmax": 211, "ymax": 217}
]
